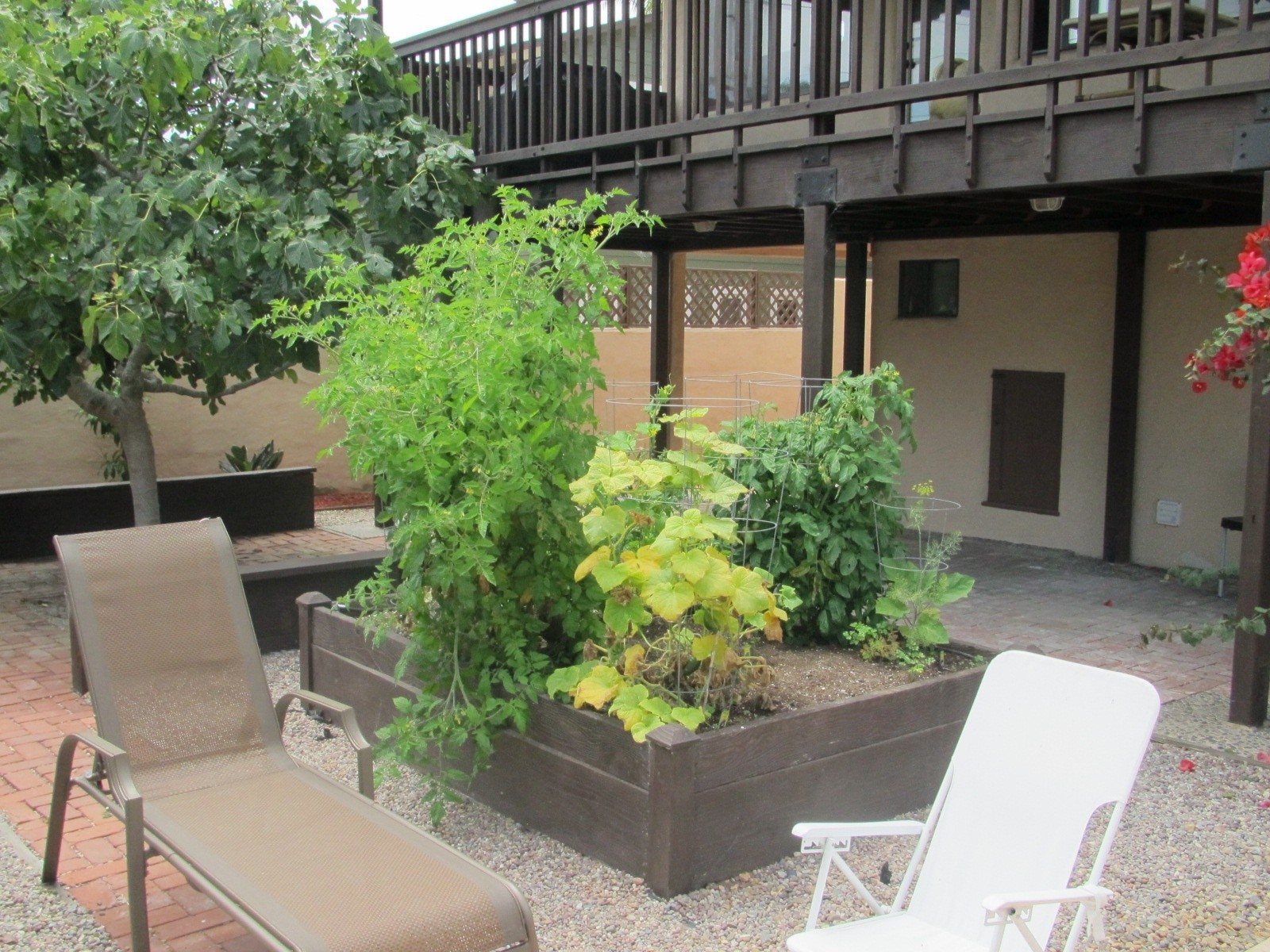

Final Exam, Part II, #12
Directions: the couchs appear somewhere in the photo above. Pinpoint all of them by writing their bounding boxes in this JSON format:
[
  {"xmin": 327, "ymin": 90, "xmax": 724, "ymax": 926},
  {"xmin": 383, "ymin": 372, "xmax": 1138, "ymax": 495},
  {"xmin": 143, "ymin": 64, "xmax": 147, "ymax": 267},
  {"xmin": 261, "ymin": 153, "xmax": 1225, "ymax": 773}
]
[{"xmin": 474, "ymin": 60, "xmax": 670, "ymax": 181}]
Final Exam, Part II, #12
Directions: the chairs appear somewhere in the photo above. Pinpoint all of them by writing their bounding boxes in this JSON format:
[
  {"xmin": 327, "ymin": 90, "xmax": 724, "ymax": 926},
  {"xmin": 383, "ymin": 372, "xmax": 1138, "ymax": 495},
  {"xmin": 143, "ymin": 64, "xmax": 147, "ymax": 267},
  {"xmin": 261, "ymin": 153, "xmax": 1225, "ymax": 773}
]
[
  {"xmin": 785, "ymin": 649, "xmax": 1162, "ymax": 952},
  {"xmin": 41, "ymin": 516, "xmax": 537, "ymax": 952}
]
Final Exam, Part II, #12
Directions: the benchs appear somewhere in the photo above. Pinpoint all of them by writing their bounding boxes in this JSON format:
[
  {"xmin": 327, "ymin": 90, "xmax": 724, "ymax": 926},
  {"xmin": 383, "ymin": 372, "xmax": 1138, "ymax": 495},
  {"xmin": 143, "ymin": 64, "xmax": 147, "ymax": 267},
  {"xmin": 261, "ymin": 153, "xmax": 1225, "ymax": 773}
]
[{"xmin": 239, "ymin": 549, "xmax": 393, "ymax": 655}]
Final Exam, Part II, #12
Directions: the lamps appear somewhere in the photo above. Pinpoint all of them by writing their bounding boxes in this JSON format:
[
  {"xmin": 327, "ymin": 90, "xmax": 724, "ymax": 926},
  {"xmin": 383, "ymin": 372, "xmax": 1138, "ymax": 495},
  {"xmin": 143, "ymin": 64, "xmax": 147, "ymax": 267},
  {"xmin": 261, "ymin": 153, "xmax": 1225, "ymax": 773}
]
[
  {"xmin": 1029, "ymin": 197, "xmax": 1065, "ymax": 212},
  {"xmin": 693, "ymin": 220, "xmax": 718, "ymax": 233}
]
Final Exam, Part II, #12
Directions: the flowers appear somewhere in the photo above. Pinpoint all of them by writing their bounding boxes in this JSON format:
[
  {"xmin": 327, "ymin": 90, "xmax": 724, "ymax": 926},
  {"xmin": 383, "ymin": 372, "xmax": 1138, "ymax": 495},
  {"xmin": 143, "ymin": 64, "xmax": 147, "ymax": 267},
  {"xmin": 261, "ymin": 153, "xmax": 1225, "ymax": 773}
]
[{"xmin": 1166, "ymin": 227, "xmax": 1270, "ymax": 396}]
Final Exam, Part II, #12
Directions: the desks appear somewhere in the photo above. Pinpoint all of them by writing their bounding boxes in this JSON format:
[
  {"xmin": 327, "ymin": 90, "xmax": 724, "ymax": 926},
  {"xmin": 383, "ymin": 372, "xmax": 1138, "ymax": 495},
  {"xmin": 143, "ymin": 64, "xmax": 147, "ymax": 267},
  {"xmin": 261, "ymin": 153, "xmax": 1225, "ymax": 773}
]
[
  {"xmin": 1217, "ymin": 516, "xmax": 1243, "ymax": 597},
  {"xmin": 1061, "ymin": 3, "xmax": 1239, "ymax": 102}
]
